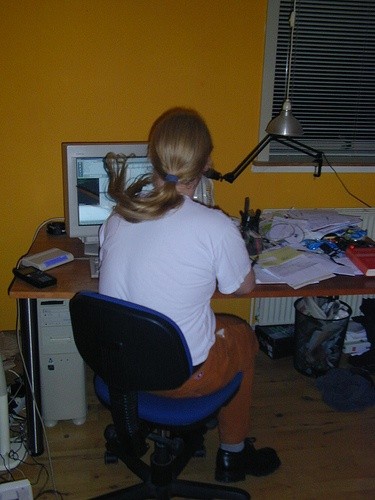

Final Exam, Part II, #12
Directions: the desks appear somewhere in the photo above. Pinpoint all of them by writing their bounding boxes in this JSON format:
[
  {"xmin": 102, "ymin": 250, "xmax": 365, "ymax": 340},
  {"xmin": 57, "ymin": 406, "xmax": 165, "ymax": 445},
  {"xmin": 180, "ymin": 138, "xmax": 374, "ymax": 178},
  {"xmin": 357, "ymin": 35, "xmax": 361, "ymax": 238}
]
[{"xmin": 8, "ymin": 224, "xmax": 375, "ymax": 457}]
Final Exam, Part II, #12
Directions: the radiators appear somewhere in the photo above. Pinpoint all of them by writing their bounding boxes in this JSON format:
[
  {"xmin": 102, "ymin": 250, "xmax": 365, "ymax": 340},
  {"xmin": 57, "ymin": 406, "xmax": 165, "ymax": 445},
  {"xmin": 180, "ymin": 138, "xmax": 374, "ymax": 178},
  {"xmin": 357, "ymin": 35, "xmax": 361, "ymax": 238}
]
[{"xmin": 254, "ymin": 207, "xmax": 375, "ymax": 326}]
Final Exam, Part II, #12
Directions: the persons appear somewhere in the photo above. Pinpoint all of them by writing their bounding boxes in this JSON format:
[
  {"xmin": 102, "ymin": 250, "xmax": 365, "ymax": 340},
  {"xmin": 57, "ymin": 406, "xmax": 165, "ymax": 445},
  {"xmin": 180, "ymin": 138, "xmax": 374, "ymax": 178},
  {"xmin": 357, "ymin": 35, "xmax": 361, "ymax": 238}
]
[{"xmin": 98, "ymin": 107, "xmax": 280, "ymax": 483}]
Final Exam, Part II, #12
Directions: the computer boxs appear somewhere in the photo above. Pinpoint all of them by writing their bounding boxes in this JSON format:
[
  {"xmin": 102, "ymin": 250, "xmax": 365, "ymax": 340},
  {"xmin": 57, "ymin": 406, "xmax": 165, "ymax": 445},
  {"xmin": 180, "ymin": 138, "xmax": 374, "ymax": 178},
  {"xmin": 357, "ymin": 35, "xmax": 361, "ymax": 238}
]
[{"xmin": 36, "ymin": 298, "xmax": 87, "ymax": 428}]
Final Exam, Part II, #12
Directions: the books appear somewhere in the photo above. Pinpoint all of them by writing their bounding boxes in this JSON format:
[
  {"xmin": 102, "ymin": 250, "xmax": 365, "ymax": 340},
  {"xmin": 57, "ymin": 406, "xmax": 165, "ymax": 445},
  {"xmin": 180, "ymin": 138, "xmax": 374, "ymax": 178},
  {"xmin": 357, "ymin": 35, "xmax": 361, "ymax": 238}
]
[
  {"xmin": 346, "ymin": 248, "xmax": 375, "ymax": 277},
  {"xmin": 250, "ymin": 246, "xmax": 336, "ymax": 289}
]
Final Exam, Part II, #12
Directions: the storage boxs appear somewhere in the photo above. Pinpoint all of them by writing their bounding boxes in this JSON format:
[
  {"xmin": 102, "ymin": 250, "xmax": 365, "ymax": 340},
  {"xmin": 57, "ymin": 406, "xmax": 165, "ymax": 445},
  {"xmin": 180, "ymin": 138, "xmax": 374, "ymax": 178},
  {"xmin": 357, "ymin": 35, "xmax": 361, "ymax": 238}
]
[{"xmin": 254, "ymin": 325, "xmax": 294, "ymax": 360}]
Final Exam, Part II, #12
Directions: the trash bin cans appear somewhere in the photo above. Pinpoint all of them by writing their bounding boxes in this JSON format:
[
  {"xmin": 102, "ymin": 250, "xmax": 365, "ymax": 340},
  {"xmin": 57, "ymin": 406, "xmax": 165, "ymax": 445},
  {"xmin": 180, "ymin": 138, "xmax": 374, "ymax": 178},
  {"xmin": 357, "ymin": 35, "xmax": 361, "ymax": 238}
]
[{"xmin": 293, "ymin": 295, "xmax": 353, "ymax": 377}]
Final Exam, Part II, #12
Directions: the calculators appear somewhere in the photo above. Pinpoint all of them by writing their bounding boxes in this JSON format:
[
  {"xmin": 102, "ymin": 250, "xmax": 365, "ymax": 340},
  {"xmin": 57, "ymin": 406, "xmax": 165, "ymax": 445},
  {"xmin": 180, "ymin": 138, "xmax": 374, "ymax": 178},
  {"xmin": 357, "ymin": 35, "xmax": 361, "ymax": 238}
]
[{"xmin": 13, "ymin": 266, "xmax": 57, "ymax": 288}]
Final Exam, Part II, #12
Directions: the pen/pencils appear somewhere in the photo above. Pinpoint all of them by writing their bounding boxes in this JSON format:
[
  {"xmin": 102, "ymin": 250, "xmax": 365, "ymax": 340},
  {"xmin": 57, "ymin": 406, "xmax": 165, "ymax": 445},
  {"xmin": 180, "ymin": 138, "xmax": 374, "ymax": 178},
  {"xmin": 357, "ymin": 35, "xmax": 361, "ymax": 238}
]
[
  {"xmin": 251, "ymin": 256, "xmax": 259, "ymax": 268},
  {"xmin": 239, "ymin": 198, "xmax": 261, "ymax": 224}
]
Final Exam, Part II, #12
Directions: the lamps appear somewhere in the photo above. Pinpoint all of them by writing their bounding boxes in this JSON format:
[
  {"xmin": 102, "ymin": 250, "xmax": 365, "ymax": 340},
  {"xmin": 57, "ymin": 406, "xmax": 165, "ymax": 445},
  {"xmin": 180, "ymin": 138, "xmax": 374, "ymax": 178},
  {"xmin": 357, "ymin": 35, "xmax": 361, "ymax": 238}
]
[{"xmin": 264, "ymin": 0, "xmax": 304, "ymax": 137}]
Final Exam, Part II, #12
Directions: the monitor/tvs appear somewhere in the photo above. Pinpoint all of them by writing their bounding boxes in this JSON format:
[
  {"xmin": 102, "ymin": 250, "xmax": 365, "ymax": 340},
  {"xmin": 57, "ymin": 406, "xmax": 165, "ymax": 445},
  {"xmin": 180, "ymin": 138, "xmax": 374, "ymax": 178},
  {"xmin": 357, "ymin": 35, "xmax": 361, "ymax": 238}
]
[{"xmin": 61, "ymin": 142, "xmax": 207, "ymax": 255}]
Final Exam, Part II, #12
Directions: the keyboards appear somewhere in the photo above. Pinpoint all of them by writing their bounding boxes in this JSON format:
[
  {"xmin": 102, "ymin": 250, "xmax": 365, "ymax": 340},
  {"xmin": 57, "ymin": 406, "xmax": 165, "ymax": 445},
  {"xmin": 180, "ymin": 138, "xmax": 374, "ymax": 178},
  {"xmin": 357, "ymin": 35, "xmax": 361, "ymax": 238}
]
[{"xmin": 90, "ymin": 257, "xmax": 100, "ymax": 279}]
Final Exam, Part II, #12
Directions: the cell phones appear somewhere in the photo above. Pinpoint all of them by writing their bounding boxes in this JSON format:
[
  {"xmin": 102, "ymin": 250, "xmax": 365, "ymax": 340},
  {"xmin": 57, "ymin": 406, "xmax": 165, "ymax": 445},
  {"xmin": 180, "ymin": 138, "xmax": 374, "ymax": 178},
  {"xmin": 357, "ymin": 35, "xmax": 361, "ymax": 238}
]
[{"xmin": 14, "ymin": 266, "xmax": 57, "ymax": 289}]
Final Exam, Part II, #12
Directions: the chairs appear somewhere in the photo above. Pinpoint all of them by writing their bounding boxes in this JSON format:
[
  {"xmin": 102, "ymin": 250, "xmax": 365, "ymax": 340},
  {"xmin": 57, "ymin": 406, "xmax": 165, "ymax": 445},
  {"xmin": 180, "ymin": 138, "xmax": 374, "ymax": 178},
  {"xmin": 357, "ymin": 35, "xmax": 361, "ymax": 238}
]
[{"xmin": 69, "ymin": 290, "xmax": 251, "ymax": 500}]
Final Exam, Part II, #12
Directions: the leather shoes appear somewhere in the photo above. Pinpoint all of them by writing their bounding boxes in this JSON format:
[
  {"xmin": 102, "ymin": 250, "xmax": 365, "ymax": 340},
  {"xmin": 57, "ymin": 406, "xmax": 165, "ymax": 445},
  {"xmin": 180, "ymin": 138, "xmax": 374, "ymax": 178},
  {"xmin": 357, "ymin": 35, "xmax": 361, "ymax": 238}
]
[
  {"xmin": 104, "ymin": 423, "xmax": 147, "ymax": 463},
  {"xmin": 215, "ymin": 437, "xmax": 281, "ymax": 482}
]
